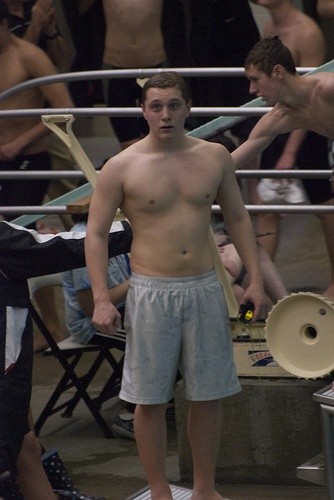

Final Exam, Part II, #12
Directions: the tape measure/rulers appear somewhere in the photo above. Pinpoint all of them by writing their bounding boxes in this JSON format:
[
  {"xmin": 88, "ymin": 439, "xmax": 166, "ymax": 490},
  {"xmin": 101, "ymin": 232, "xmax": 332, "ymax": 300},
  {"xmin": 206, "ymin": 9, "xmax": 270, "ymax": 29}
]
[{"xmin": 238, "ymin": 302, "xmax": 255, "ymax": 324}]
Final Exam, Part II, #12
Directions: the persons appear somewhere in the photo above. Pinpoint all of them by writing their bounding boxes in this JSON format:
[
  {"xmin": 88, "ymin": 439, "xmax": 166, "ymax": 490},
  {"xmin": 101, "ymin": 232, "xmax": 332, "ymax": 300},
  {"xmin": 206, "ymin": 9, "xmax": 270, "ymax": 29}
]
[
  {"xmin": 83, "ymin": 75, "xmax": 264, "ymax": 500},
  {"xmin": 0, "ymin": 0, "xmax": 334, "ymax": 500}
]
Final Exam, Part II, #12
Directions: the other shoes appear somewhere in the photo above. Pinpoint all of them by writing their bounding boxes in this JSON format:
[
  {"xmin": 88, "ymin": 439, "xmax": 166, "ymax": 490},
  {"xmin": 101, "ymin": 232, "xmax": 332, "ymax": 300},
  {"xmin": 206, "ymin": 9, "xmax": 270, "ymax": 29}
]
[
  {"xmin": 112, "ymin": 416, "xmax": 136, "ymax": 441},
  {"xmin": 166, "ymin": 405, "xmax": 175, "ymax": 428}
]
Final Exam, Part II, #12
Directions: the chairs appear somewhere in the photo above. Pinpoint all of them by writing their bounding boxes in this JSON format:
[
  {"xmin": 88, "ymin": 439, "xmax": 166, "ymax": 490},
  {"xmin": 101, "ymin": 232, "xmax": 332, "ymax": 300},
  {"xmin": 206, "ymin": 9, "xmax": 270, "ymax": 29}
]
[{"xmin": 27, "ymin": 273, "xmax": 124, "ymax": 439}]
